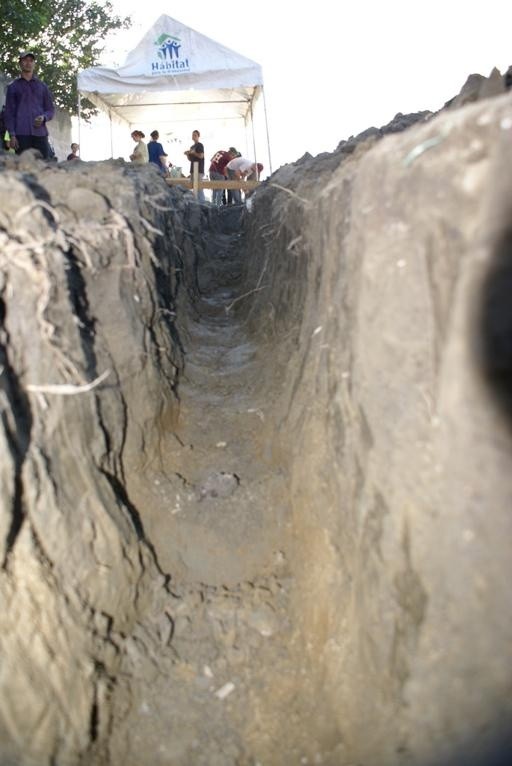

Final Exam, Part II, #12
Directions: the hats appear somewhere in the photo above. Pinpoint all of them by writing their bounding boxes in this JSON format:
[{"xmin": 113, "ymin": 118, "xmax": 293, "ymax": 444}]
[{"xmin": 20, "ymin": 52, "xmax": 35, "ymax": 60}]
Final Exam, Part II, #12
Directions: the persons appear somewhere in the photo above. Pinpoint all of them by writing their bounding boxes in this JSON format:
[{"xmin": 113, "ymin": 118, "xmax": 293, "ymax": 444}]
[
  {"xmin": 4, "ymin": 126, "xmax": 16, "ymax": 155},
  {"xmin": 208, "ymin": 147, "xmax": 238, "ymax": 207},
  {"xmin": 67, "ymin": 142, "xmax": 80, "ymax": 162},
  {"xmin": 129, "ymin": 129, "xmax": 149, "ymax": 163},
  {"xmin": 221, "ymin": 151, "xmax": 242, "ymax": 207},
  {"xmin": 146, "ymin": 130, "xmax": 168, "ymax": 175},
  {"xmin": 6, "ymin": 50, "xmax": 55, "ymax": 162},
  {"xmin": 224, "ymin": 156, "xmax": 264, "ymax": 206},
  {"xmin": 184, "ymin": 129, "xmax": 206, "ymax": 202}
]
